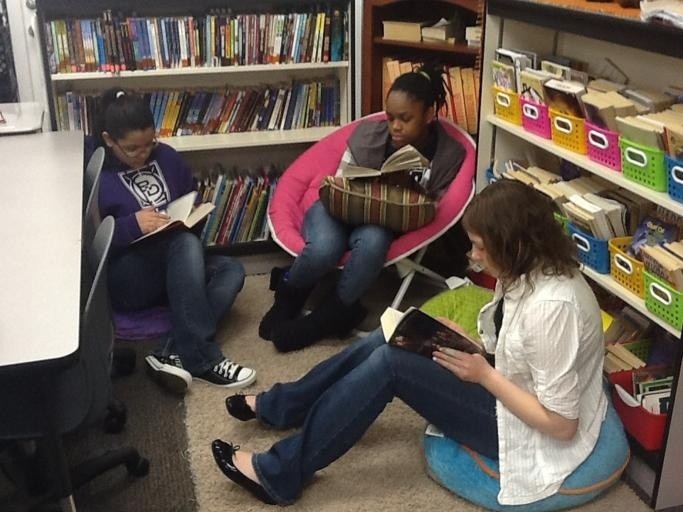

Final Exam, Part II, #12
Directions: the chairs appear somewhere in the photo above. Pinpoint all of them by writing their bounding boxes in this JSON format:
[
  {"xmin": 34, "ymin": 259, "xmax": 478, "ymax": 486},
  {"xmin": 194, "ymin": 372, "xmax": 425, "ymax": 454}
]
[
  {"xmin": 79, "ymin": 149, "xmax": 133, "ymax": 429},
  {"xmin": 266, "ymin": 112, "xmax": 477, "ymax": 339},
  {"xmin": 0, "ymin": 212, "xmax": 149, "ymax": 511}
]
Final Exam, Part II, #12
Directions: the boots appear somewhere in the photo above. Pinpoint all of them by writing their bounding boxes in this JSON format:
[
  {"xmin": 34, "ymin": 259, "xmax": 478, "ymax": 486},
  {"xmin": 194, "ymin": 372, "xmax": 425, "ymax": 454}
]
[
  {"xmin": 259, "ymin": 266, "xmax": 314, "ymax": 340},
  {"xmin": 271, "ymin": 285, "xmax": 366, "ymax": 352}
]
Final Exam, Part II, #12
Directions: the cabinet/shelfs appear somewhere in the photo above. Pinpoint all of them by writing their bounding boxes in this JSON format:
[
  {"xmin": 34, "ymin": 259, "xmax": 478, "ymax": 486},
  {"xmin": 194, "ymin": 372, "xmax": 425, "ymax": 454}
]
[
  {"xmin": 21, "ymin": 1, "xmax": 356, "ymax": 264},
  {"xmin": 477, "ymin": 0, "xmax": 683, "ymax": 512},
  {"xmin": 361, "ymin": 0, "xmax": 486, "ymax": 164}
]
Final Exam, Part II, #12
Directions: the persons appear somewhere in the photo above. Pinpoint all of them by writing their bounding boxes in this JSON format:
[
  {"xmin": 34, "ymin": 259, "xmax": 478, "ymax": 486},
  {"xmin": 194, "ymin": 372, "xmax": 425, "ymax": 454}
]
[
  {"xmin": 211, "ymin": 178, "xmax": 607, "ymax": 508},
  {"xmin": 84, "ymin": 89, "xmax": 256, "ymax": 396},
  {"xmin": 258, "ymin": 64, "xmax": 466, "ymax": 352}
]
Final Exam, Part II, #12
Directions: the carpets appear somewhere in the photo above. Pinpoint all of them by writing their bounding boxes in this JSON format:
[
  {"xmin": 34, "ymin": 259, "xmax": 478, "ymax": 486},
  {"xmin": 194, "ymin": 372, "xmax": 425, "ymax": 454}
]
[{"xmin": 181, "ymin": 275, "xmax": 653, "ymax": 512}]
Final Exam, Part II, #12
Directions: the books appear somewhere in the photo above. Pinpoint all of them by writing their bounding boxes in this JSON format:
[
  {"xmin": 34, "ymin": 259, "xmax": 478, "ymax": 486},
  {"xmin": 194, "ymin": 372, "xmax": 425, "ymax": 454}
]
[
  {"xmin": 382, "ymin": 13, "xmax": 469, "ymax": 43},
  {"xmin": 443, "ymin": 62, "xmax": 481, "ymax": 133},
  {"xmin": 45, "ymin": 1, "xmax": 345, "ymax": 73},
  {"xmin": 492, "ymin": 48, "xmax": 683, "ymax": 161},
  {"xmin": 378, "ymin": 53, "xmax": 422, "ymax": 110},
  {"xmin": 126, "ymin": 190, "xmax": 216, "ymax": 248},
  {"xmin": 378, "ymin": 303, "xmax": 484, "ymax": 362},
  {"xmin": 191, "ymin": 162, "xmax": 286, "ymax": 245},
  {"xmin": 342, "ymin": 145, "xmax": 432, "ymax": 183},
  {"xmin": 492, "ymin": 143, "xmax": 683, "ymax": 301},
  {"xmin": 56, "ymin": 74, "xmax": 341, "ymax": 138},
  {"xmin": 601, "ymin": 302, "xmax": 676, "ymax": 416}
]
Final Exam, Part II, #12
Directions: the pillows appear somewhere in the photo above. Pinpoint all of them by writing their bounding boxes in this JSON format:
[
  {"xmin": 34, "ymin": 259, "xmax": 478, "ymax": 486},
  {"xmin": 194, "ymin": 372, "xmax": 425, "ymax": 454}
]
[{"xmin": 319, "ymin": 176, "xmax": 435, "ymax": 233}]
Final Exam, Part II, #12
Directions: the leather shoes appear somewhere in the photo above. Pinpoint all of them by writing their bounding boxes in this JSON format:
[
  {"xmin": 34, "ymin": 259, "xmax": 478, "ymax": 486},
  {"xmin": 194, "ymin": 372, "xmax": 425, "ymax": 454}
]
[
  {"xmin": 212, "ymin": 439, "xmax": 278, "ymax": 505},
  {"xmin": 226, "ymin": 391, "xmax": 256, "ymax": 421}
]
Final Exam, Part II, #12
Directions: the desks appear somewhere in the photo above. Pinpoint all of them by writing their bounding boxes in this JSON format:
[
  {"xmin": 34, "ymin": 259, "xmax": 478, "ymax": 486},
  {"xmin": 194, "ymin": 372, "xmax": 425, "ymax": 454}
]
[{"xmin": 0, "ymin": 131, "xmax": 86, "ymax": 370}]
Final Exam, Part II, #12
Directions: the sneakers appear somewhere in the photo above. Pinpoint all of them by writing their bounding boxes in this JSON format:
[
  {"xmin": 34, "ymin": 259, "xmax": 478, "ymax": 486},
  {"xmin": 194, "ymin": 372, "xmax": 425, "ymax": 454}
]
[
  {"xmin": 192, "ymin": 357, "xmax": 257, "ymax": 389},
  {"xmin": 144, "ymin": 352, "xmax": 193, "ymax": 395}
]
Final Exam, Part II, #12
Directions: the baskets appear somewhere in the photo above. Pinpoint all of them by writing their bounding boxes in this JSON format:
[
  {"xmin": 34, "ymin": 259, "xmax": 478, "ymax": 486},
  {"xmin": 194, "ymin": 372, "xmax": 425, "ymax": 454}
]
[
  {"xmin": 485, "ymin": 167, "xmax": 683, "ymax": 331},
  {"xmin": 490, "ymin": 84, "xmax": 683, "ymax": 205},
  {"xmin": 605, "ymin": 331, "xmax": 656, "ymax": 365}
]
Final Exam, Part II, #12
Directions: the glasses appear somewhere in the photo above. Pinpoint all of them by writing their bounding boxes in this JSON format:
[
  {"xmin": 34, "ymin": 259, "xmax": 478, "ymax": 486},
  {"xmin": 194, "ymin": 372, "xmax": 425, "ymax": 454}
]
[{"xmin": 110, "ymin": 135, "xmax": 160, "ymax": 159}]
